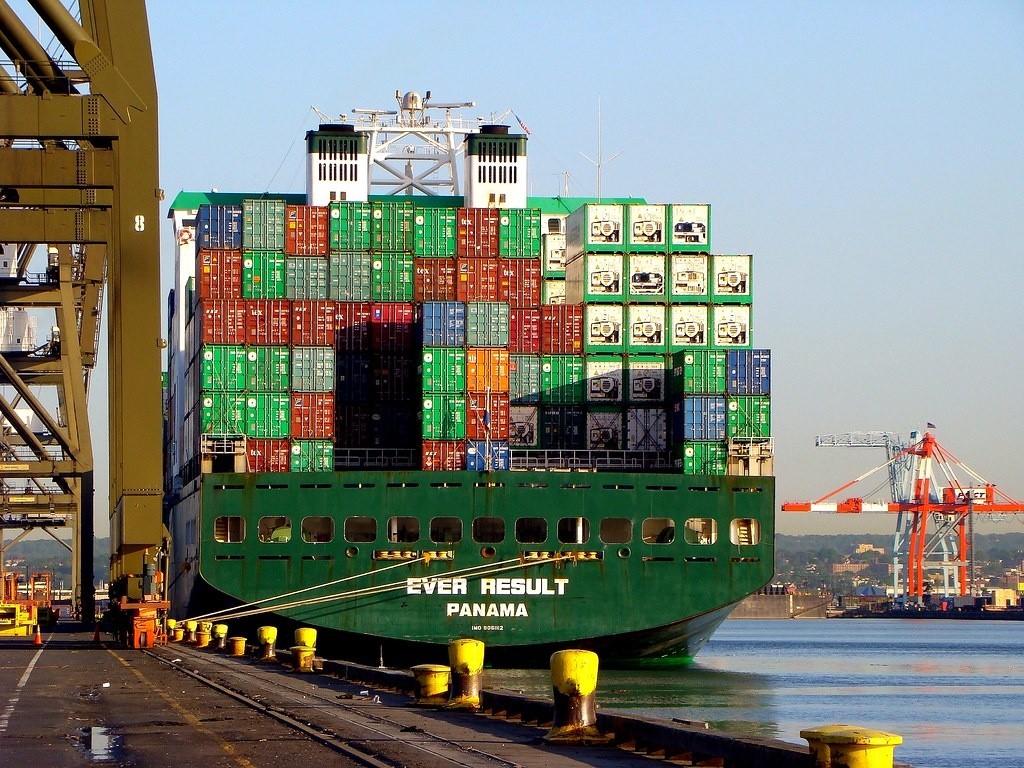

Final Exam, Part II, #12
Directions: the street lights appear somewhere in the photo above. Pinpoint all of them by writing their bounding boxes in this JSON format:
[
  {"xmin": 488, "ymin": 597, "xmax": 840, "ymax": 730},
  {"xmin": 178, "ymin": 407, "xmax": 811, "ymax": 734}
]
[{"xmin": 44, "ymin": 561, "xmax": 63, "ymax": 600}]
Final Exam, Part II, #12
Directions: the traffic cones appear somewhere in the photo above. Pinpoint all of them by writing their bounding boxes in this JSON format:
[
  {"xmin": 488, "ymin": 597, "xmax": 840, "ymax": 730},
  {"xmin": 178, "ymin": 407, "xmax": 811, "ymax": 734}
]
[
  {"xmin": 32, "ymin": 623, "xmax": 45, "ymax": 645},
  {"xmin": 93, "ymin": 625, "xmax": 102, "ymax": 643}
]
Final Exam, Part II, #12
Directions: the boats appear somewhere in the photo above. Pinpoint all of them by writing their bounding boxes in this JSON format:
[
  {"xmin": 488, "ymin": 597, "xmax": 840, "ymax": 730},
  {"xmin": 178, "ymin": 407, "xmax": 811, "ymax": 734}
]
[{"xmin": 163, "ymin": 92, "xmax": 775, "ymax": 665}]
